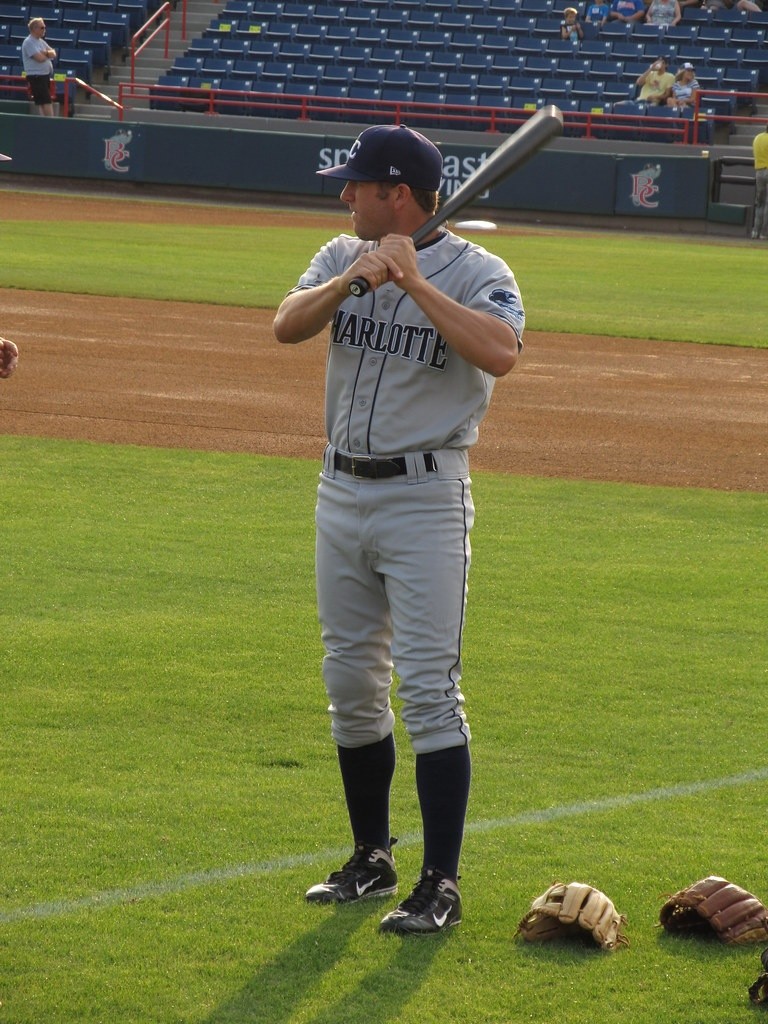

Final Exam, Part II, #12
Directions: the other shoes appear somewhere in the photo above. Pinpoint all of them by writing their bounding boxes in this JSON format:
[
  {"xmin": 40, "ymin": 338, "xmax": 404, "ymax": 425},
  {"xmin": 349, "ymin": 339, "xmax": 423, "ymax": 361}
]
[{"xmin": 751, "ymin": 231, "xmax": 768, "ymax": 239}]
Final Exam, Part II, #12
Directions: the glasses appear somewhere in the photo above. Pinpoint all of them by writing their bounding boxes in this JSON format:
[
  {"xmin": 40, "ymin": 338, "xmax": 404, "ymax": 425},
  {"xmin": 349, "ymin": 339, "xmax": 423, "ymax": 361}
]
[{"xmin": 40, "ymin": 26, "xmax": 46, "ymax": 29}]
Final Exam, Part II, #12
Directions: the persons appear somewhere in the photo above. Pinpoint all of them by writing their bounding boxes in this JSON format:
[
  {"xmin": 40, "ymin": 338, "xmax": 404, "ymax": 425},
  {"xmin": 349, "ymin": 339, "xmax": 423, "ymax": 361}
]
[
  {"xmin": 610, "ymin": 0, "xmax": 644, "ymax": 23},
  {"xmin": 585, "ymin": 0, "xmax": 609, "ymax": 23},
  {"xmin": 668, "ymin": 63, "xmax": 699, "ymax": 107},
  {"xmin": 559, "ymin": 8, "xmax": 583, "ymax": 40},
  {"xmin": 621, "ymin": 55, "xmax": 675, "ymax": 106},
  {"xmin": 22, "ymin": 18, "xmax": 56, "ymax": 116},
  {"xmin": 751, "ymin": 125, "xmax": 768, "ymax": 240},
  {"xmin": 682, "ymin": 0, "xmax": 761, "ymax": 11},
  {"xmin": 644, "ymin": 0, "xmax": 682, "ymax": 25},
  {"xmin": 274, "ymin": 124, "xmax": 525, "ymax": 934}
]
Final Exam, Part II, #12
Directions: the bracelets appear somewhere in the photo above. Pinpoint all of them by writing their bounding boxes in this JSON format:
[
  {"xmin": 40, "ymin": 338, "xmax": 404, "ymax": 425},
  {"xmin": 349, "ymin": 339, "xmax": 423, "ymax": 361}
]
[{"xmin": 45, "ymin": 51, "xmax": 48, "ymax": 58}]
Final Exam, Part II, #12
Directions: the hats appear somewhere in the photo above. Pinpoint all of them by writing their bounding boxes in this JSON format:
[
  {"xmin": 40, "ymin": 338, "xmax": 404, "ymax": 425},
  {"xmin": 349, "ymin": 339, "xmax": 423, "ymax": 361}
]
[
  {"xmin": 679, "ymin": 62, "xmax": 696, "ymax": 71},
  {"xmin": 316, "ymin": 123, "xmax": 443, "ymax": 191}
]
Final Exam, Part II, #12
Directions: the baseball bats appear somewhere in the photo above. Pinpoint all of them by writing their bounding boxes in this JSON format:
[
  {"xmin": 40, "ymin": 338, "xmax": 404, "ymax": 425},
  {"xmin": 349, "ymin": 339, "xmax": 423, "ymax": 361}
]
[{"xmin": 346, "ymin": 103, "xmax": 569, "ymax": 301}]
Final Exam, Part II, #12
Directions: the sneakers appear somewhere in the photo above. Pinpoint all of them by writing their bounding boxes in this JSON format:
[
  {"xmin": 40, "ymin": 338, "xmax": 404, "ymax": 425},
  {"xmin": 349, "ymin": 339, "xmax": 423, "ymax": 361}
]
[
  {"xmin": 306, "ymin": 837, "xmax": 399, "ymax": 906},
  {"xmin": 379, "ymin": 864, "xmax": 463, "ymax": 937}
]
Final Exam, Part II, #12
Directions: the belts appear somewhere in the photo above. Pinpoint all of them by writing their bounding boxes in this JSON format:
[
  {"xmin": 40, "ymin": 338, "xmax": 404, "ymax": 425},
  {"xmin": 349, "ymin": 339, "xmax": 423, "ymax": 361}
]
[{"xmin": 334, "ymin": 453, "xmax": 438, "ymax": 479}]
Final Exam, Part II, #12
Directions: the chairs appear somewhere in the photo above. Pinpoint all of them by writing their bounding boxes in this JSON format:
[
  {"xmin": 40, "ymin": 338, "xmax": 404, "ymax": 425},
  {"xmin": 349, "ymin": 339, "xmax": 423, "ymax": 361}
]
[{"xmin": 0, "ymin": 0, "xmax": 768, "ymax": 148}]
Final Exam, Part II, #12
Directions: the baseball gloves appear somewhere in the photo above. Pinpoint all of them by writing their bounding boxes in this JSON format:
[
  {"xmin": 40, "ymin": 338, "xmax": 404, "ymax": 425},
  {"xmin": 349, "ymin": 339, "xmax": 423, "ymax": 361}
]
[
  {"xmin": 658, "ymin": 873, "xmax": 767, "ymax": 947},
  {"xmin": 518, "ymin": 880, "xmax": 623, "ymax": 950}
]
